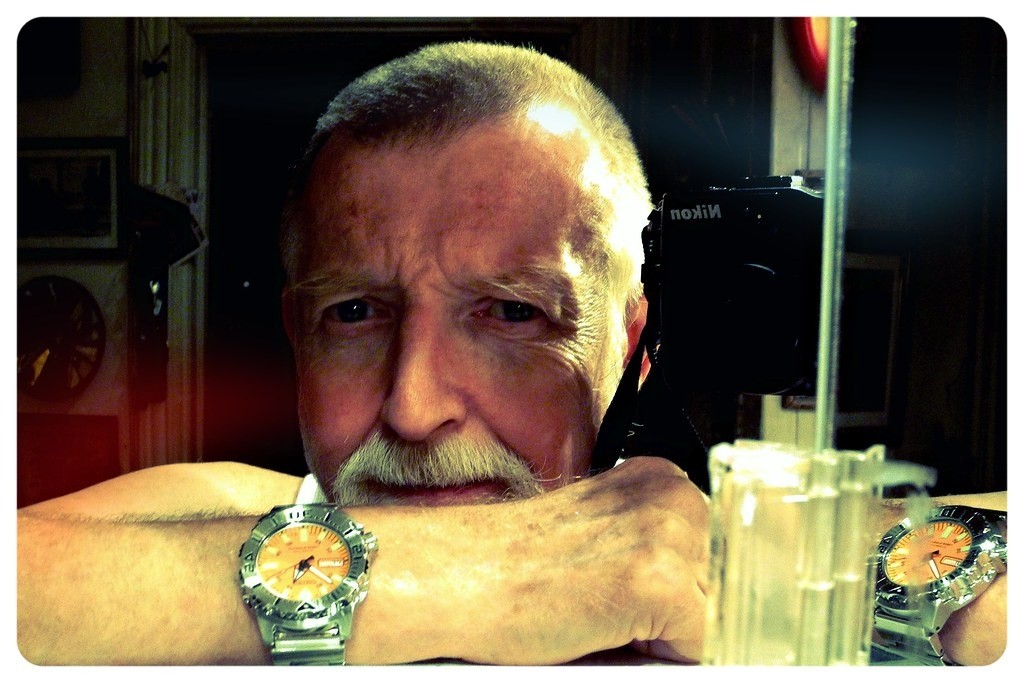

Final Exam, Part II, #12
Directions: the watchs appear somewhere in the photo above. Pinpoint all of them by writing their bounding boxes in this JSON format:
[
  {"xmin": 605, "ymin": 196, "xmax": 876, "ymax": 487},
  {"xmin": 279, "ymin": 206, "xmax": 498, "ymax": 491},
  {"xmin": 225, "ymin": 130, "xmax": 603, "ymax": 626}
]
[
  {"xmin": 869, "ymin": 506, "xmax": 1008, "ymax": 666},
  {"xmin": 238, "ymin": 504, "xmax": 378, "ymax": 666}
]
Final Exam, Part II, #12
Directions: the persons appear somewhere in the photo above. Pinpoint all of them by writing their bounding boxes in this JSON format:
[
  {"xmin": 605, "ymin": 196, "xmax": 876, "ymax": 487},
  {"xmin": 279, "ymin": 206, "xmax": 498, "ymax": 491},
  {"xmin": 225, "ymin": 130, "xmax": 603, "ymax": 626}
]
[{"xmin": 17, "ymin": 42, "xmax": 1007, "ymax": 666}]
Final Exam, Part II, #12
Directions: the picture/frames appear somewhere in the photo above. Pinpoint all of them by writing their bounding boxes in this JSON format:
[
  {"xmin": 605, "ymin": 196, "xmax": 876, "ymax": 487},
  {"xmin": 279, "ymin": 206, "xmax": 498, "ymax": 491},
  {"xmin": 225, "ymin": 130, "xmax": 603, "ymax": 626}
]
[
  {"xmin": 784, "ymin": 247, "xmax": 909, "ymax": 428},
  {"xmin": 17, "ymin": 136, "xmax": 133, "ymax": 259}
]
